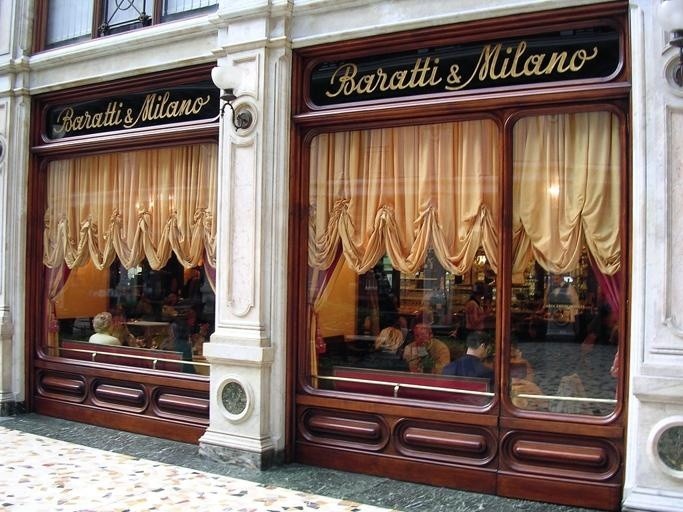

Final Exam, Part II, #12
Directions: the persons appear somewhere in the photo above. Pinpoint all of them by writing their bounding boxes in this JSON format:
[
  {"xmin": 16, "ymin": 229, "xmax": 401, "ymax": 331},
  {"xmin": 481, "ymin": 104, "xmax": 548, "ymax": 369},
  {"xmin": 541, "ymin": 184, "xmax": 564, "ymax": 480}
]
[
  {"xmin": 363, "ymin": 261, "xmax": 580, "ymax": 378},
  {"xmin": 159, "ymin": 321, "xmax": 196, "ymax": 374},
  {"xmin": 89, "ymin": 312, "xmax": 120, "ymax": 345}
]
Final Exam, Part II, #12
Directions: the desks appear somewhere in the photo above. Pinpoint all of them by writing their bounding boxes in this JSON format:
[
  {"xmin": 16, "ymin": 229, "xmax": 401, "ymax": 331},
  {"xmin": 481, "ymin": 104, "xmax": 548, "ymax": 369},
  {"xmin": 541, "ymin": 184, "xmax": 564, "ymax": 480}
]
[
  {"xmin": 349, "ymin": 335, "xmax": 380, "ymax": 354},
  {"xmin": 123, "ymin": 320, "xmax": 170, "ymax": 349}
]
